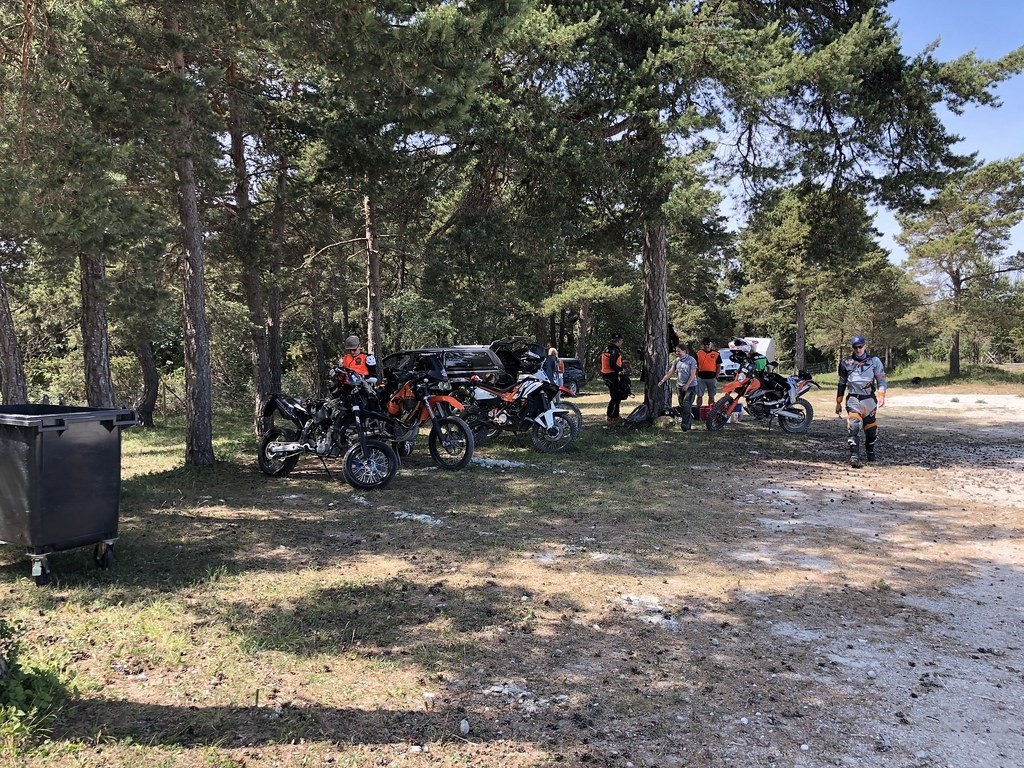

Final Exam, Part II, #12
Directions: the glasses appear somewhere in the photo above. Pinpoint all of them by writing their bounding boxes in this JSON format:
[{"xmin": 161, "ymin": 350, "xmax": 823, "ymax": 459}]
[
  {"xmin": 854, "ymin": 344, "xmax": 865, "ymax": 350},
  {"xmin": 348, "ymin": 347, "xmax": 359, "ymax": 352},
  {"xmin": 704, "ymin": 343, "xmax": 710, "ymax": 346}
]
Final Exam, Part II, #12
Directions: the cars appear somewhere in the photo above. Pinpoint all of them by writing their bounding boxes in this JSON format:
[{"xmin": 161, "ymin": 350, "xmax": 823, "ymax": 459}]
[{"xmin": 716, "ymin": 350, "xmax": 741, "ymax": 382}]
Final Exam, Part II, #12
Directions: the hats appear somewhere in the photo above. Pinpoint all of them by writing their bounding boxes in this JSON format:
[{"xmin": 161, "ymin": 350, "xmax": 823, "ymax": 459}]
[
  {"xmin": 611, "ymin": 333, "xmax": 624, "ymax": 339},
  {"xmin": 851, "ymin": 336, "xmax": 865, "ymax": 347},
  {"xmin": 345, "ymin": 336, "xmax": 360, "ymax": 350},
  {"xmin": 702, "ymin": 337, "xmax": 712, "ymax": 343}
]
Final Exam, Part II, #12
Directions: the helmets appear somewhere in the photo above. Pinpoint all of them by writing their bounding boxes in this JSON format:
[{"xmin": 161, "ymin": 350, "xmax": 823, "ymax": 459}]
[{"xmin": 730, "ymin": 337, "xmax": 751, "ymax": 354}]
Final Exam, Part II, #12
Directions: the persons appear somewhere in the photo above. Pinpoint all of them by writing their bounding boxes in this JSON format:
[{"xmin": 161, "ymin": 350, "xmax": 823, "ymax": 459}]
[
  {"xmin": 339, "ymin": 335, "xmax": 377, "ymax": 383},
  {"xmin": 601, "ymin": 336, "xmax": 626, "ymax": 424},
  {"xmin": 542, "ymin": 347, "xmax": 564, "ymax": 403},
  {"xmin": 750, "ymin": 349, "xmax": 770, "ymax": 372},
  {"xmin": 836, "ymin": 336, "xmax": 887, "ymax": 467},
  {"xmin": 658, "ymin": 344, "xmax": 698, "ymax": 431},
  {"xmin": 695, "ymin": 337, "xmax": 724, "ymax": 411}
]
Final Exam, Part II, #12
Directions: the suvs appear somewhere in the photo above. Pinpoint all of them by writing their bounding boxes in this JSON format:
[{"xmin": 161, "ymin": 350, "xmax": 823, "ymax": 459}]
[
  {"xmin": 382, "ymin": 334, "xmax": 527, "ymax": 397},
  {"xmin": 502, "ymin": 349, "xmax": 583, "ymax": 398}
]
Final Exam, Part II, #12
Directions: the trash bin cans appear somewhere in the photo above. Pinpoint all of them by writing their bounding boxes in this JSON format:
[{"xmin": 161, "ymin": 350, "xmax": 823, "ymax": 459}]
[
  {"xmin": 752, "ymin": 354, "xmax": 768, "ymax": 371},
  {"xmin": 0, "ymin": 403, "xmax": 140, "ymax": 586}
]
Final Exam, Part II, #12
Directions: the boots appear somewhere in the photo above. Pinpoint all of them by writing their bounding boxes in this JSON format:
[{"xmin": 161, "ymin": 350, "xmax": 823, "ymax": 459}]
[
  {"xmin": 847, "ymin": 436, "xmax": 859, "ymax": 467},
  {"xmin": 865, "ymin": 436, "xmax": 877, "ymax": 462}
]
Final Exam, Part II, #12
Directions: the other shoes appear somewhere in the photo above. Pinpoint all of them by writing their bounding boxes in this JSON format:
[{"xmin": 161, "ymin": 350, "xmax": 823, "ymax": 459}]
[
  {"xmin": 606, "ymin": 416, "xmax": 615, "ymax": 424},
  {"xmin": 681, "ymin": 424, "xmax": 687, "ymax": 432}
]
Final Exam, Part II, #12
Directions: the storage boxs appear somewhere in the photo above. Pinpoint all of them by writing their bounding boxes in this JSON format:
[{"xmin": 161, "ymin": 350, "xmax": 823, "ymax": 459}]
[{"xmin": 700, "ymin": 403, "xmax": 744, "ymax": 422}]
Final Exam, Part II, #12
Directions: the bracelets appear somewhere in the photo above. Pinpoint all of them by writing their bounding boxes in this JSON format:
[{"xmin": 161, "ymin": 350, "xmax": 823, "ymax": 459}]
[{"xmin": 661, "ymin": 380, "xmax": 664, "ymax": 382}]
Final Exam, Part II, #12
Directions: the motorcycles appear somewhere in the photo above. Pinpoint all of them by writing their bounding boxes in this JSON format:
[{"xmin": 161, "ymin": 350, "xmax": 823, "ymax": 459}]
[
  {"xmin": 255, "ymin": 364, "xmax": 398, "ymax": 491},
  {"xmin": 705, "ymin": 350, "xmax": 823, "ymax": 435},
  {"xmin": 446, "ymin": 351, "xmax": 584, "ymax": 454},
  {"xmin": 341, "ymin": 367, "xmax": 474, "ymax": 473}
]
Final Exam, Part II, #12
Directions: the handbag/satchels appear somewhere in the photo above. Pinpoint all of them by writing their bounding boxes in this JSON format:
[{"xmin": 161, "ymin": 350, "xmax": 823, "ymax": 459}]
[{"xmin": 618, "ymin": 373, "xmax": 632, "ymax": 400}]
